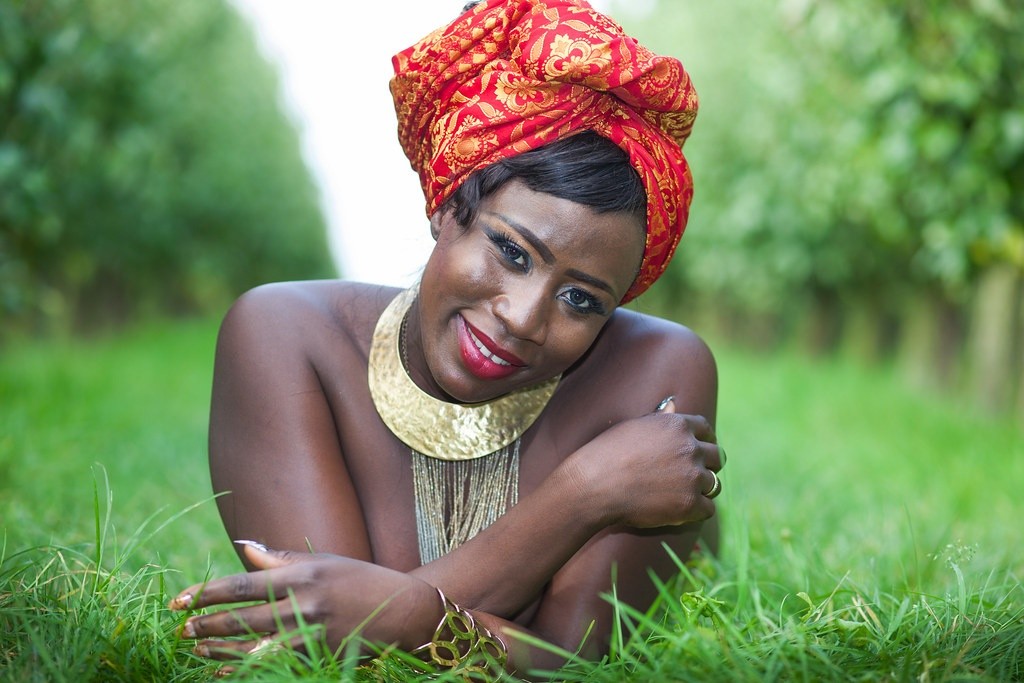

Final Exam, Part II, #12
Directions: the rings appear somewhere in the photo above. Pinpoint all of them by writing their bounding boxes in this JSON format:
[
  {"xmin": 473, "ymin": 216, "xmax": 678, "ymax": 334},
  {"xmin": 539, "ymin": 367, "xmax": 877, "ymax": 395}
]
[{"xmin": 705, "ymin": 470, "xmax": 718, "ymax": 497}]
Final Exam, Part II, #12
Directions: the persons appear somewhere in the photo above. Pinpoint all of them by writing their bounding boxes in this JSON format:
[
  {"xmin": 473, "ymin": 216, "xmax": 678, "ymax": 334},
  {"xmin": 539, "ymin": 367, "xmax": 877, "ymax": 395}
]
[{"xmin": 169, "ymin": 0, "xmax": 728, "ymax": 683}]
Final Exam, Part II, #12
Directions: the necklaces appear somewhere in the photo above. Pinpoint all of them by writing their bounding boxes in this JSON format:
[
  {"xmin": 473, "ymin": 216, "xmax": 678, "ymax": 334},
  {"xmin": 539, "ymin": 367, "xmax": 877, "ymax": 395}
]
[{"xmin": 368, "ymin": 282, "xmax": 563, "ymax": 566}]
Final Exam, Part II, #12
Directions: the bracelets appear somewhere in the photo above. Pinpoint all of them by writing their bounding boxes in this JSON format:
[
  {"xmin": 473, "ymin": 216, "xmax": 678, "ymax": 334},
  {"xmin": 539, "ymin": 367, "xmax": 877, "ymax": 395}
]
[{"xmin": 407, "ymin": 586, "xmax": 508, "ymax": 683}]
